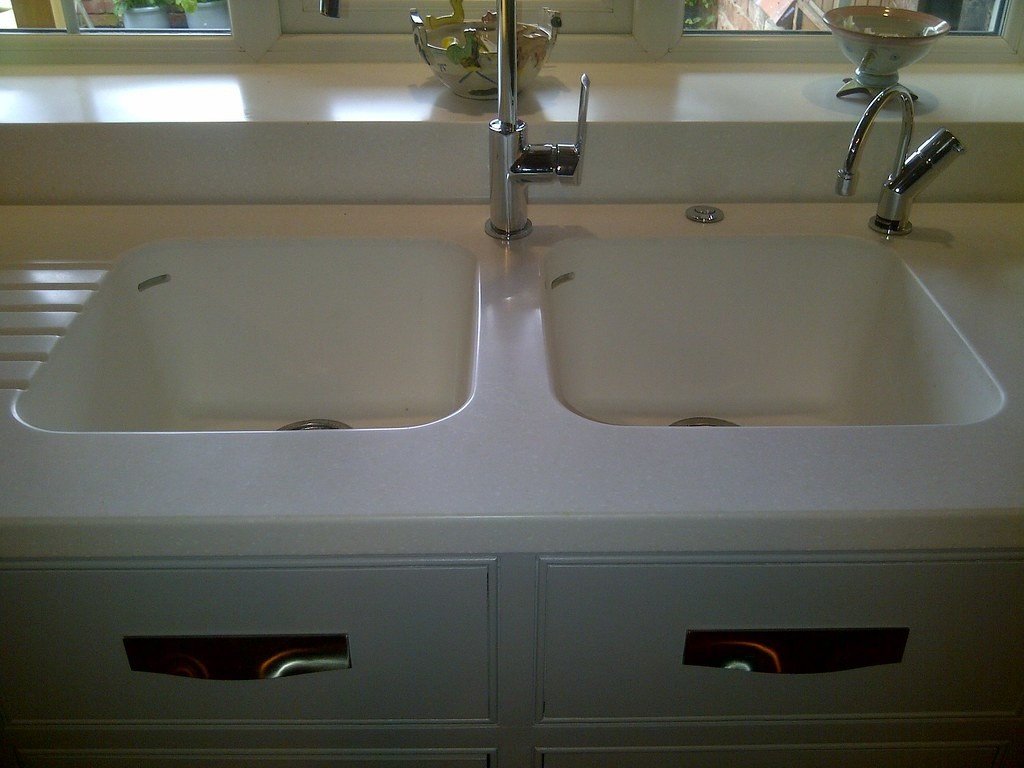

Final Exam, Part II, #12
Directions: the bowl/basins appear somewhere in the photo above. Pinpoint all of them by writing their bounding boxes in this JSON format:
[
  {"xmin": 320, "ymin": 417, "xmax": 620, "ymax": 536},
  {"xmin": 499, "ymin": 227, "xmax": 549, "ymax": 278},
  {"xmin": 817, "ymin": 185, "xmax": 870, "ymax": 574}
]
[
  {"xmin": 408, "ymin": 0, "xmax": 562, "ymax": 101},
  {"xmin": 822, "ymin": 5, "xmax": 950, "ymax": 102}
]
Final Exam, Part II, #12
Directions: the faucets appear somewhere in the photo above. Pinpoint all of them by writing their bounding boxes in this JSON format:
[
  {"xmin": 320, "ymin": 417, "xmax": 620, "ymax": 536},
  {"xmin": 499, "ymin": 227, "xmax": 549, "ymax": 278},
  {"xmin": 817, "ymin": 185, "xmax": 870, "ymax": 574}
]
[
  {"xmin": 315, "ymin": 0, "xmax": 594, "ymax": 243},
  {"xmin": 834, "ymin": 83, "xmax": 966, "ymax": 238}
]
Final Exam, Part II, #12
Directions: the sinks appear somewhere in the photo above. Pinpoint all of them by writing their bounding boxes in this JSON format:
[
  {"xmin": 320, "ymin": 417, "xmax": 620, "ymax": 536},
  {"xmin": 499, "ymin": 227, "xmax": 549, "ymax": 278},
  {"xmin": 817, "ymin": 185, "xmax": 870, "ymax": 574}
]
[
  {"xmin": 10, "ymin": 203, "xmax": 553, "ymax": 438},
  {"xmin": 471, "ymin": 201, "xmax": 1007, "ymax": 431}
]
